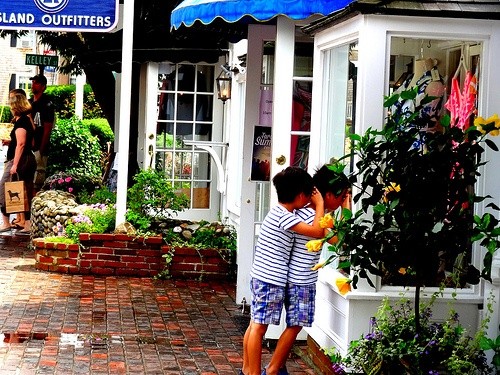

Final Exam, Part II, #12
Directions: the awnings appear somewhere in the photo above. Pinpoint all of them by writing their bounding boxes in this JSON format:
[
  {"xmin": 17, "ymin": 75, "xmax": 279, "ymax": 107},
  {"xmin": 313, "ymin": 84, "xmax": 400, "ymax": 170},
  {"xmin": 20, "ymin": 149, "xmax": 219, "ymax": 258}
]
[{"xmin": 169, "ymin": 0, "xmax": 357, "ymax": 34}]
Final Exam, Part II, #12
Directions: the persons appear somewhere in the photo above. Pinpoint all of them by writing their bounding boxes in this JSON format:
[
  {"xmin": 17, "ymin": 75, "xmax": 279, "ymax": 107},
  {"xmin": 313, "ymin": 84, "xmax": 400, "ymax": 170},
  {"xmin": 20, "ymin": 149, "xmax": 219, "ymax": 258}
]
[
  {"xmin": 389, "ymin": 57, "xmax": 445, "ymax": 156},
  {"xmin": 0, "ymin": 87, "xmax": 37, "ymax": 236},
  {"xmin": 240, "ymin": 166, "xmax": 324, "ymax": 375},
  {"xmin": 261, "ymin": 165, "xmax": 349, "ymax": 375},
  {"xmin": 7, "ymin": 90, "xmax": 35, "ymax": 231},
  {"xmin": 11, "ymin": 73, "xmax": 55, "ymax": 229}
]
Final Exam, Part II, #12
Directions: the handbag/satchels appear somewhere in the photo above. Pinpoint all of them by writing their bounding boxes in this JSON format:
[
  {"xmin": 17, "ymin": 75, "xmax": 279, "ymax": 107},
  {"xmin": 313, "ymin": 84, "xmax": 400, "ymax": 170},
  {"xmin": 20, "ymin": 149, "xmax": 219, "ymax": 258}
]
[{"xmin": 4, "ymin": 172, "xmax": 29, "ymax": 213}]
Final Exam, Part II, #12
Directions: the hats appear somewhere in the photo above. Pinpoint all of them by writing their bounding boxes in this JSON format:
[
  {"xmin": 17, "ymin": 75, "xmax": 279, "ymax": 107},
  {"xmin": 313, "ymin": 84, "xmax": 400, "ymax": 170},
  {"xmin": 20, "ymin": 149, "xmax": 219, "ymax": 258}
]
[{"xmin": 29, "ymin": 74, "xmax": 47, "ymax": 85}]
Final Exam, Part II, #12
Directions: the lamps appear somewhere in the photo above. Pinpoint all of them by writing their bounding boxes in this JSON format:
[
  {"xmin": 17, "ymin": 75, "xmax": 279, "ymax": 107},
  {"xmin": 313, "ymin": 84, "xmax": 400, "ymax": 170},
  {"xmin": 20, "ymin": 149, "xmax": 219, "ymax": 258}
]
[{"xmin": 215, "ymin": 62, "xmax": 232, "ymax": 105}]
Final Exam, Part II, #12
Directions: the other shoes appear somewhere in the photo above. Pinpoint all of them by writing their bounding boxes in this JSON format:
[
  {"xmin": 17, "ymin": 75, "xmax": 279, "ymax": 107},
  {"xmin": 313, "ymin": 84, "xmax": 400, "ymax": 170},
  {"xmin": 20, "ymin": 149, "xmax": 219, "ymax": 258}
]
[{"xmin": 0, "ymin": 217, "xmax": 32, "ymax": 236}]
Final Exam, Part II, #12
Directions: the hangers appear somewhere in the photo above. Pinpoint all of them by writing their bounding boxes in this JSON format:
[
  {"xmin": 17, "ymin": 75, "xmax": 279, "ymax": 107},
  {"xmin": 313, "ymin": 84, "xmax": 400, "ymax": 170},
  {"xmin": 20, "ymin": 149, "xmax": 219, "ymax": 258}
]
[{"xmin": 420, "ymin": 39, "xmax": 468, "ymax": 80}]
